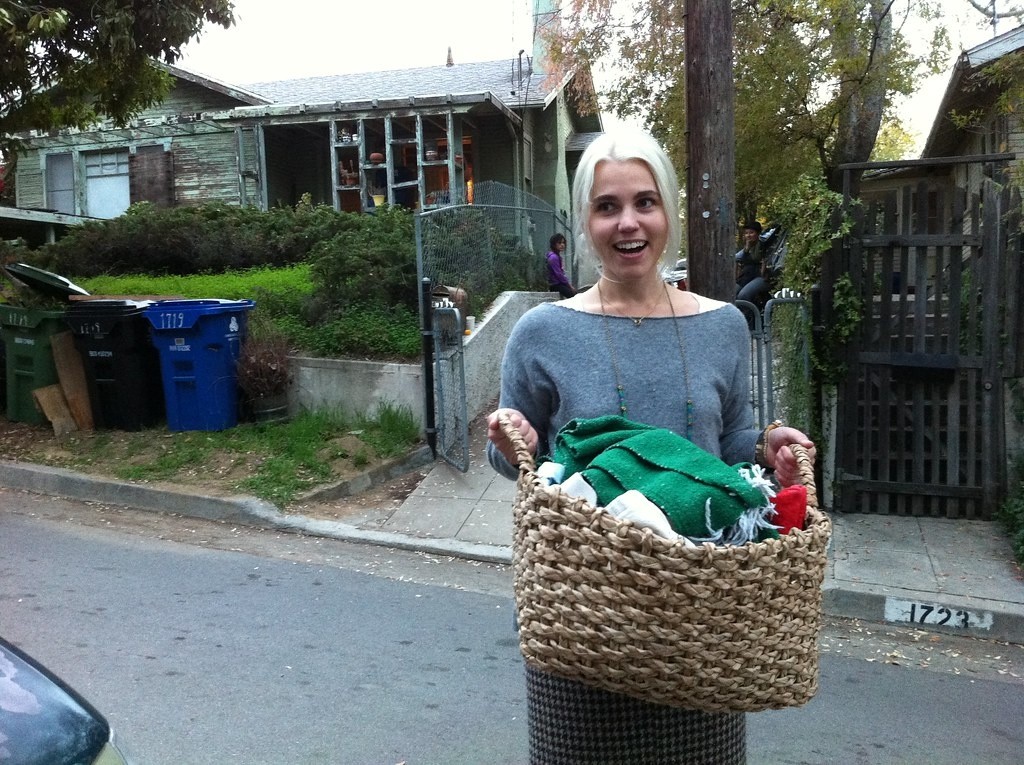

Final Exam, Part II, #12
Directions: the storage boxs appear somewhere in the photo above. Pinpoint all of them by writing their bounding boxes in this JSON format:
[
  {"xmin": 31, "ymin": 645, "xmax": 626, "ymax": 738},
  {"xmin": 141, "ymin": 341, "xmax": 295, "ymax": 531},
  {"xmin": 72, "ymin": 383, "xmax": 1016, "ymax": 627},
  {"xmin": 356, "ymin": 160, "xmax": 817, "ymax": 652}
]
[{"xmin": 495, "ymin": 411, "xmax": 834, "ymax": 712}]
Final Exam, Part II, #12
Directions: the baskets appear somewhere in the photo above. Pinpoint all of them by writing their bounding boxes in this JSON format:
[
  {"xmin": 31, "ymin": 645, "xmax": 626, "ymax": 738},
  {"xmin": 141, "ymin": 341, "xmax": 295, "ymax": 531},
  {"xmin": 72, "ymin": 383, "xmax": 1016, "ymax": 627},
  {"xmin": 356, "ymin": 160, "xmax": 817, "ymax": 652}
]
[{"xmin": 497, "ymin": 407, "xmax": 832, "ymax": 712}]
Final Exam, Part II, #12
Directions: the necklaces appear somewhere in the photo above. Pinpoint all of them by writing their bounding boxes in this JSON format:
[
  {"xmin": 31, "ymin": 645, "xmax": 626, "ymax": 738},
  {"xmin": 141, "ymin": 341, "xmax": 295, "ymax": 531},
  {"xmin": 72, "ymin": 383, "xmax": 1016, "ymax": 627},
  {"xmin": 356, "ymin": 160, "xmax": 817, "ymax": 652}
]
[
  {"xmin": 597, "ymin": 276, "xmax": 664, "ymax": 324},
  {"xmin": 599, "ymin": 279, "xmax": 692, "ymax": 444}
]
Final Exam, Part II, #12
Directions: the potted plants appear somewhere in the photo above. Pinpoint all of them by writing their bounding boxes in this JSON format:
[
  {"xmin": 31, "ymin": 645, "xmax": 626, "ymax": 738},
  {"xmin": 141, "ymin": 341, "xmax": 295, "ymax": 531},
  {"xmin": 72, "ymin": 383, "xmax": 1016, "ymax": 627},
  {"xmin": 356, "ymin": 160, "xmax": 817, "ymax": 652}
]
[
  {"xmin": 341, "ymin": 127, "xmax": 352, "ymax": 144},
  {"xmin": 368, "ymin": 179, "xmax": 386, "ymax": 207},
  {"xmin": 425, "ymin": 184, "xmax": 438, "ymax": 204},
  {"xmin": 338, "ymin": 158, "xmax": 358, "ymax": 186}
]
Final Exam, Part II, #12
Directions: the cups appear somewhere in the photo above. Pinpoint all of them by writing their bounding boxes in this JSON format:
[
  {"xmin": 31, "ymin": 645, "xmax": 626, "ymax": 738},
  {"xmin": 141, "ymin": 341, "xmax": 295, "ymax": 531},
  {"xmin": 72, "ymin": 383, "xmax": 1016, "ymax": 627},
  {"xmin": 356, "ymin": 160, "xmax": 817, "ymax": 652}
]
[
  {"xmin": 372, "ymin": 195, "xmax": 385, "ymax": 206},
  {"xmin": 465, "ymin": 316, "xmax": 475, "ymax": 333}
]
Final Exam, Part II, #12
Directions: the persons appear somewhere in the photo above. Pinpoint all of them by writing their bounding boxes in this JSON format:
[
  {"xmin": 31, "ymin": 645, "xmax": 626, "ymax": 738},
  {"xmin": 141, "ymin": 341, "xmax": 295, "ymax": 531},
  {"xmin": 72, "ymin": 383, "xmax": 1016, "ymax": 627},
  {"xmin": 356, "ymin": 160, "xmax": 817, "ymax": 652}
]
[
  {"xmin": 546, "ymin": 234, "xmax": 578, "ymax": 298},
  {"xmin": 488, "ymin": 129, "xmax": 815, "ymax": 765},
  {"xmin": 739, "ymin": 221, "xmax": 764, "ymax": 287}
]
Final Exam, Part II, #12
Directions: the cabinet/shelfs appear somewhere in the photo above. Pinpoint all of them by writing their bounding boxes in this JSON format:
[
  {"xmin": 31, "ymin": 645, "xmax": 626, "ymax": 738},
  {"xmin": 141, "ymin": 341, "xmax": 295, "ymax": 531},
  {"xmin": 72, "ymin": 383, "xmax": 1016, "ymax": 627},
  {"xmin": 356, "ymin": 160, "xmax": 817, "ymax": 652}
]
[{"xmin": 328, "ymin": 105, "xmax": 465, "ymax": 223}]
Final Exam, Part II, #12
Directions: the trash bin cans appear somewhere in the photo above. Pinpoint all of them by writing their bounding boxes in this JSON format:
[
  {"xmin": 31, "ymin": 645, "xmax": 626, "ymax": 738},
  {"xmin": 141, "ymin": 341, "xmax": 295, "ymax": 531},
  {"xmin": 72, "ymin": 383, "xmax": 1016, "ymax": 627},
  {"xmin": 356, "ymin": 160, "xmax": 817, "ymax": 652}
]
[
  {"xmin": 143, "ymin": 296, "xmax": 257, "ymax": 431},
  {"xmin": 0, "ymin": 262, "xmax": 91, "ymax": 426},
  {"xmin": 65, "ymin": 297, "xmax": 167, "ymax": 432}
]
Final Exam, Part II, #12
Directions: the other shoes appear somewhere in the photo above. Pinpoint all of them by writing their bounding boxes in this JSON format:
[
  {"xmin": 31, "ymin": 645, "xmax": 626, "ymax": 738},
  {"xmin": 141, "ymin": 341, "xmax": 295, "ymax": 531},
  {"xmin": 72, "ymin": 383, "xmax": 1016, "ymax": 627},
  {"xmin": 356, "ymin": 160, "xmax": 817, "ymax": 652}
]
[{"xmin": 748, "ymin": 319, "xmax": 757, "ymax": 331}]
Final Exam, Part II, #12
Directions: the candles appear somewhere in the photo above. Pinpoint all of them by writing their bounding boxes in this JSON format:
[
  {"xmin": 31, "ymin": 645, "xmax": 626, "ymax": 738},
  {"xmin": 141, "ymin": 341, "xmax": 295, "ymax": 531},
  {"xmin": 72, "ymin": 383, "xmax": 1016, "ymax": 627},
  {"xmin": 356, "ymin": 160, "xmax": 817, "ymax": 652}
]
[{"xmin": 425, "ymin": 140, "xmax": 438, "ymax": 160}]
